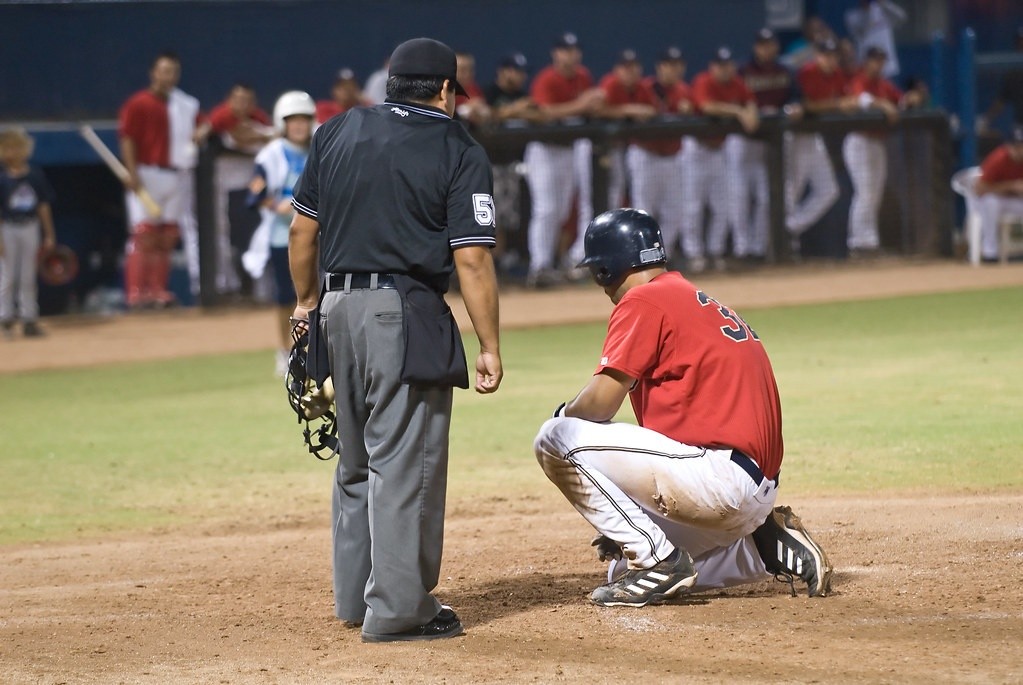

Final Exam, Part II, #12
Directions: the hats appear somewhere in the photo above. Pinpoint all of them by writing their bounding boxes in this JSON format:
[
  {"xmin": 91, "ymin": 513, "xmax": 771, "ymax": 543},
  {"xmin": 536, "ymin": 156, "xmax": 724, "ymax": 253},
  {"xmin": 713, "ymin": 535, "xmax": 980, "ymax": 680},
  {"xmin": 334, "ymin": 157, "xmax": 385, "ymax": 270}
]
[
  {"xmin": 615, "ymin": 47, "xmax": 640, "ymax": 64},
  {"xmin": 816, "ymin": 39, "xmax": 838, "ymax": 53},
  {"xmin": 756, "ymin": 28, "xmax": 773, "ymax": 42},
  {"xmin": 389, "ymin": 38, "xmax": 470, "ymax": 99},
  {"xmin": 866, "ymin": 47, "xmax": 888, "ymax": 59},
  {"xmin": 709, "ymin": 46, "xmax": 734, "ymax": 61},
  {"xmin": 660, "ymin": 46, "xmax": 684, "ymax": 64},
  {"xmin": 556, "ymin": 32, "xmax": 582, "ymax": 53},
  {"xmin": 499, "ymin": 51, "xmax": 530, "ymax": 72}
]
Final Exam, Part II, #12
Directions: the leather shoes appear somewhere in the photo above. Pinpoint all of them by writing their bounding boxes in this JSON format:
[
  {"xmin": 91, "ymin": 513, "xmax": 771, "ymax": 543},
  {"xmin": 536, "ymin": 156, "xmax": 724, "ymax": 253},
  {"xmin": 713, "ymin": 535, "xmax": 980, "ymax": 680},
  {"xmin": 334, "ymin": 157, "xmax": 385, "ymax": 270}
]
[{"xmin": 360, "ymin": 608, "xmax": 463, "ymax": 641}]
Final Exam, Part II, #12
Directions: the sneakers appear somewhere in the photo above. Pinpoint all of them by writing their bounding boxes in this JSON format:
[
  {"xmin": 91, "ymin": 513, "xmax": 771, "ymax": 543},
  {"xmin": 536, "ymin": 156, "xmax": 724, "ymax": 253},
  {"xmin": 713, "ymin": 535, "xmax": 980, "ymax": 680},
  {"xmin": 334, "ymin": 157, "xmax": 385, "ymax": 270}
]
[
  {"xmin": 587, "ymin": 547, "xmax": 697, "ymax": 607},
  {"xmin": 754, "ymin": 505, "xmax": 830, "ymax": 598}
]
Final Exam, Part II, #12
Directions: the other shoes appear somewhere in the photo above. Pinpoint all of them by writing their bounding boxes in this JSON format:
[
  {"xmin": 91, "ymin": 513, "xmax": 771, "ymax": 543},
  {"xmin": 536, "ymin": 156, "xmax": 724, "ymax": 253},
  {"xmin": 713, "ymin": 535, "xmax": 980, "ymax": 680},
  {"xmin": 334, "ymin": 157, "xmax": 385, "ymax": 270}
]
[{"xmin": 274, "ymin": 351, "xmax": 291, "ymax": 374}]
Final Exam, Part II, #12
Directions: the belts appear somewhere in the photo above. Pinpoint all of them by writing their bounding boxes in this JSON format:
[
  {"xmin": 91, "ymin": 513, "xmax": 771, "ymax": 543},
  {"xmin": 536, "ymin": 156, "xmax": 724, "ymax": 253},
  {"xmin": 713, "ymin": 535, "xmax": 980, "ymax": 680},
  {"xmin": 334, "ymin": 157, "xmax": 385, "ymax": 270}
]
[
  {"xmin": 731, "ymin": 448, "xmax": 780, "ymax": 489},
  {"xmin": 330, "ymin": 272, "xmax": 397, "ymax": 291}
]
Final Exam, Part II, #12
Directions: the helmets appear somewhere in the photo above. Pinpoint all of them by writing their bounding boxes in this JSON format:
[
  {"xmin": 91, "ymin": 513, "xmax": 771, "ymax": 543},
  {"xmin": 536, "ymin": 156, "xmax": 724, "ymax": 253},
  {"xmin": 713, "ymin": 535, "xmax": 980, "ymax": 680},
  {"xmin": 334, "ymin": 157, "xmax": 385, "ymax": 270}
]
[
  {"xmin": 272, "ymin": 91, "xmax": 316, "ymax": 137},
  {"xmin": 575, "ymin": 208, "xmax": 666, "ymax": 286}
]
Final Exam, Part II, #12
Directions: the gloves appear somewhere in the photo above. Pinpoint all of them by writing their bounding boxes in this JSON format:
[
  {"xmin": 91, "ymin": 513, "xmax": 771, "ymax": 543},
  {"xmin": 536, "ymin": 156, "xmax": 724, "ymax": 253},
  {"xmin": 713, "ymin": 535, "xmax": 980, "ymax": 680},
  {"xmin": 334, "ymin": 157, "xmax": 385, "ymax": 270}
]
[
  {"xmin": 591, "ymin": 534, "xmax": 622, "ymax": 561},
  {"xmin": 553, "ymin": 402, "xmax": 567, "ymax": 417}
]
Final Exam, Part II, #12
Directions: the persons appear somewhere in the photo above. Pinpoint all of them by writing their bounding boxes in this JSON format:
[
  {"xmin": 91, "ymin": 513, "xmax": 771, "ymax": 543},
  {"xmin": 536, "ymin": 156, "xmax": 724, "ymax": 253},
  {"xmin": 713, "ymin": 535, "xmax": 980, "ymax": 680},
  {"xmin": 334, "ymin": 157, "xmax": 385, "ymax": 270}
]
[
  {"xmin": 534, "ymin": 208, "xmax": 831, "ymax": 608},
  {"xmin": 313, "ymin": 66, "xmax": 374, "ymax": 124},
  {"xmin": 455, "ymin": 50, "xmax": 493, "ymax": 124},
  {"xmin": 487, "ymin": 50, "xmax": 552, "ymax": 274},
  {"xmin": 363, "ymin": 56, "xmax": 393, "ymax": 106},
  {"xmin": 119, "ymin": 48, "xmax": 208, "ymax": 312},
  {"xmin": 247, "ymin": 90, "xmax": 319, "ymax": 377},
  {"xmin": 845, "ymin": 0, "xmax": 906, "ymax": 92},
  {"xmin": 777, "ymin": 15, "xmax": 873, "ymax": 257},
  {"xmin": 727, "ymin": 26, "xmax": 803, "ymax": 261},
  {"xmin": 626, "ymin": 46, "xmax": 695, "ymax": 268},
  {"xmin": 842, "ymin": 45, "xmax": 924, "ymax": 259},
  {"xmin": 585, "ymin": 48, "xmax": 668, "ymax": 210},
  {"xmin": 973, "ymin": 127, "xmax": 1023, "ymax": 265},
  {"xmin": 524, "ymin": 32, "xmax": 604, "ymax": 287},
  {"xmin": 192, "ymin": 78, "xmax": 271, "ymax": 155},
  {"xmin": 0, "ymin": 123, "xmax": 56, "ymax": 337},
  {"xmin": 682, "ymin": 46, "xmax": 759, "ymax": 271},
  {"xmin": 288, "ymin": 37, "xmax": 502, "ymax": 641}
]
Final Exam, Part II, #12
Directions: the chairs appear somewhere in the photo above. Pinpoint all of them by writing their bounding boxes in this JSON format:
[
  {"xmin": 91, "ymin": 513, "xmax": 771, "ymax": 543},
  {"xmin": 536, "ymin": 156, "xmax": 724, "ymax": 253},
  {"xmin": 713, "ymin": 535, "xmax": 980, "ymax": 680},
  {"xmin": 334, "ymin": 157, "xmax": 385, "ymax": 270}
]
[{"xmin": 952, "ymin": 167, "xmax": 1023, "ymax": 268}]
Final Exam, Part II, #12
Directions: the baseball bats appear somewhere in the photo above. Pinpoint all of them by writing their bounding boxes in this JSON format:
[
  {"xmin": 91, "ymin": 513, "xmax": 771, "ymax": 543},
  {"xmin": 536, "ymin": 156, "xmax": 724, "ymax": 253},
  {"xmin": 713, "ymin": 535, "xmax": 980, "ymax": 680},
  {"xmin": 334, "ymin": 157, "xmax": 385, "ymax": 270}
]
[{"xmin": 80, "ymin": 124, "xmax": 162, "ymax": 216}]
